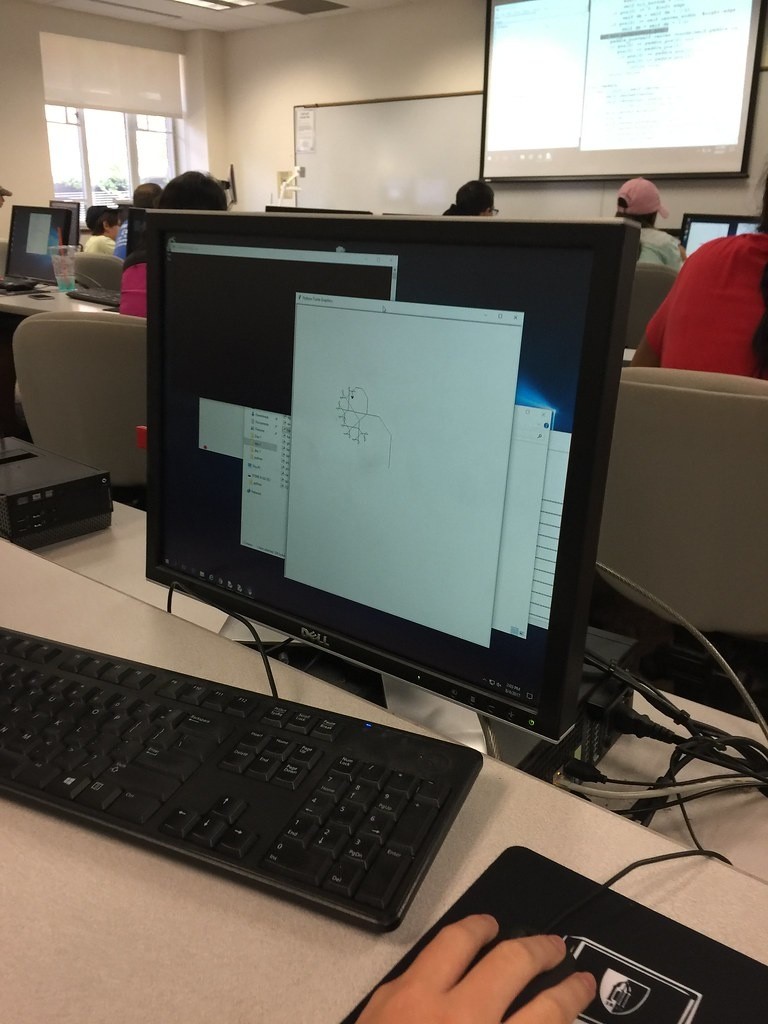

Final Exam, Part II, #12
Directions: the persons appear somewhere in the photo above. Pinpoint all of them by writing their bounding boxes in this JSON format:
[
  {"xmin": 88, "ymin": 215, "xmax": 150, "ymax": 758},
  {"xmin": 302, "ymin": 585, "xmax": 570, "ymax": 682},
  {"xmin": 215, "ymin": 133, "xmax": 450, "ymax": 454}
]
[
  {"xmin": 627, "ymin": 161, "xmax": 768, "ymax": 381},
  {"xmin": 354, "ymin": 913, "xmax": 597, "ymax": 1024},
  {"xmin": 81, "ymin": 171, "xmax": 228, "ymax": 320},
  {"xmin": 440, "ymin": 181, "xmax": 495, "ymax": 217},
  {"xmin": 610, "ymin": 177, "xmax": 686, "ymax": 277}
]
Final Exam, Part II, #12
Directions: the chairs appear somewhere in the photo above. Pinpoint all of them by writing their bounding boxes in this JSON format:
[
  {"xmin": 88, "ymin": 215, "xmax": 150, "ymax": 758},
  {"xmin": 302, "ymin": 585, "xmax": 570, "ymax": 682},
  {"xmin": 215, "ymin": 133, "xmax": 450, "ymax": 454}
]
[
  {"xmin": 625, "ymin": 261, "xmax": 677, "ymax": 348},
  {"xmin": 74, "ymin": 253, "xmax": 125, "ymax": 291},
  {"xmin": 596, "ymin": 365, "xmax": 768, "ymax": 635},
  {"xmin": 13, "ymin": 310, "xmax": 147, "ymax": 484}
]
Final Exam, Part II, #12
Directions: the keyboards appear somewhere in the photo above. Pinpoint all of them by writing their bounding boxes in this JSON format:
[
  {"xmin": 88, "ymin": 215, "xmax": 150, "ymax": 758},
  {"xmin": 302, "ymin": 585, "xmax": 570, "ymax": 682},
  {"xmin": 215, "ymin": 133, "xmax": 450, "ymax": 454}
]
[
  {"xmin": 66, "ymin": 288, "xmax": 122, "ymax": 307},
  {"xmin": 0, "ymin": 627, "xmax": 483, "ymax": 931},
  {"xmin": 0, "ymin": 277, "xmax": 38, "ymax": 292}
]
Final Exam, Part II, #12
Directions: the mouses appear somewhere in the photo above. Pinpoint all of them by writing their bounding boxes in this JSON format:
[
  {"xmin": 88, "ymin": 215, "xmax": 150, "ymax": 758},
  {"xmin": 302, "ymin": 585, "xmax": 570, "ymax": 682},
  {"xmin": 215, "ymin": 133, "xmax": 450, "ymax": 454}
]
[{"xmin": 460, "ymin": 925, "xmax": 578, "ymax": 1023}]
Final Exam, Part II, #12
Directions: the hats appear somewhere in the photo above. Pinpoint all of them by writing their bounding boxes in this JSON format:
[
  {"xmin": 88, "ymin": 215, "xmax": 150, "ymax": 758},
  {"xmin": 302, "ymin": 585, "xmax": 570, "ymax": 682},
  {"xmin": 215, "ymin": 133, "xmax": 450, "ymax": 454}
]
[
  {"xmin": 617, "ymin": 178, "xmax": 670, "ymax": 221},
  {"xmin": 83, "ymin": 205, "xmax": 124, "ymax": 231}
]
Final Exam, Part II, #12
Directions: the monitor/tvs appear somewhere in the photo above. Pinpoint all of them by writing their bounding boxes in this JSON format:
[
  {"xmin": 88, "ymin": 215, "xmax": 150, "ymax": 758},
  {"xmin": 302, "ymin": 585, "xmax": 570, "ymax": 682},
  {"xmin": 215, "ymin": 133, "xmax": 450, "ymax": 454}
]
[{"xmin": 3, "ymin": 200, "xmax": 767, "ymax": 743}]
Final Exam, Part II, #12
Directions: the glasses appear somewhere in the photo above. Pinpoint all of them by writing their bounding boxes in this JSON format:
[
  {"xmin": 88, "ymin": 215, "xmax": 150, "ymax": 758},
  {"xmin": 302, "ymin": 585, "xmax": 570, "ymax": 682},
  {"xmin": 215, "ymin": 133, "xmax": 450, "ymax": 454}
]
[{"xmin": 488, "ymin": 207, "xmax": 499, "ymax": 215}]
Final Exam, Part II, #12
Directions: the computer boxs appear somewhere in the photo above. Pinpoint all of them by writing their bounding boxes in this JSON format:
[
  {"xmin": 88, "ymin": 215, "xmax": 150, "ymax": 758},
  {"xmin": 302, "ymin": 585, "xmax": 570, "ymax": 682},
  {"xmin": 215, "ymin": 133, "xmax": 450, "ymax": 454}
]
[{"xmin": 216, "ymin": 615, "xmax": 645, "ymax": 789}]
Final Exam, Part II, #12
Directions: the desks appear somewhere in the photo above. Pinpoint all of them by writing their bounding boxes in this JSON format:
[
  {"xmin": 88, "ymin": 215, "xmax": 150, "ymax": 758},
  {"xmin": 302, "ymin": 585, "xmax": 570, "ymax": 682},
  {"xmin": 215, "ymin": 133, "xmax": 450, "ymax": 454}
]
[
  {"xmin": 0, "ymin": 502, "xmax": 768, "ymax": 1024},
  {"xmin": 0, "ymin": 274, "xmax": 123, "ymax": 315}
]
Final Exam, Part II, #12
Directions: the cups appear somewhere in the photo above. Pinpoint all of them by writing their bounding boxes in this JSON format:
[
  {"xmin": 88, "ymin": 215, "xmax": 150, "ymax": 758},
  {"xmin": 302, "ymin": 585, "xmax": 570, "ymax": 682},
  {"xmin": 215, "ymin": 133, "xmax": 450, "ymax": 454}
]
[{"xmin": 47, "ymin": 245, "xmax": 77, "ymax": 291}]
